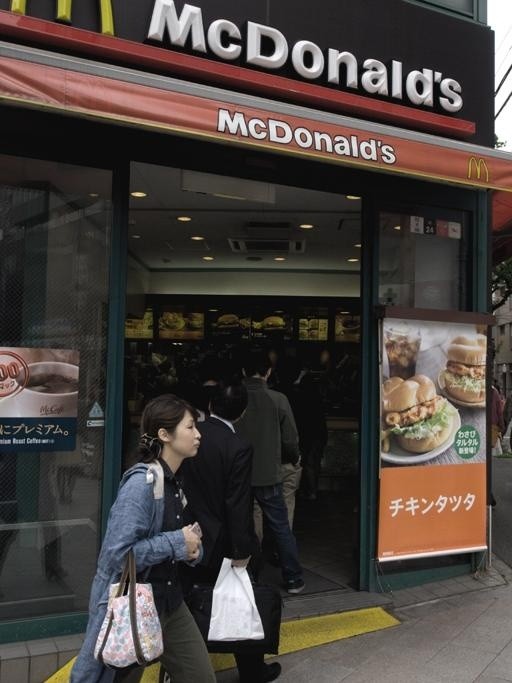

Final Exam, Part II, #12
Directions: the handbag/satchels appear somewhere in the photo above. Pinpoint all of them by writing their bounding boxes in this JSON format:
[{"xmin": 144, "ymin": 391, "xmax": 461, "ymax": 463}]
[
  {"xmin": 94, "ymin": 549, "xmax": 165, "ymax": 671},
  {"xmin": 189, "ymin": 558, "xmax": 282, "ymax": 654}
]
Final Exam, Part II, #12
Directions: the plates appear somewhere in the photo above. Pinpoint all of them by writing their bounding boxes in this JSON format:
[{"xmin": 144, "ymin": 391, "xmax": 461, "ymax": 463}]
[
  {"xmin": 380, "ymin": 396, "xmax": 461, "ymax": 466},
  {"xmin": 159, "ymin": 316, "xmax": 186, "ymax": 331},
  {"xmin": 439, "ymin": 370, "xmax": 487, "ymax": 408}
]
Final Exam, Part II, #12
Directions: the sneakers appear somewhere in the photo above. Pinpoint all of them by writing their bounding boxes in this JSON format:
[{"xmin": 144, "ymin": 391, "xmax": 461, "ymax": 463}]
[
  {"xmin": 287, "ymin": 579, "xmax": 305, "ymax": 593},
  {"xmin": 238, "ymin": 662, "xmax": 281, "ymax": 683}
]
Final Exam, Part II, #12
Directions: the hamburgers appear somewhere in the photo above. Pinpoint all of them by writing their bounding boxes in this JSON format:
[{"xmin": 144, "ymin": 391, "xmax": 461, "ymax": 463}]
[
  {"xmin": 264, "ymin": 316, "xmax": 285, "ymax": 330},
  {"xmin": 444, "ymin": 334, "xmax": 487, "ymax": 403},
  {"xmin": 217, "ymin": 314, "xmax": 240, "ymax": 329},
  {"xmin": 383, "ymin": 375, "xmax": 454, "ymax": 454},
  {"xmin": 188, "ymin": 313, "xmax": 204, "ymax": 329}
]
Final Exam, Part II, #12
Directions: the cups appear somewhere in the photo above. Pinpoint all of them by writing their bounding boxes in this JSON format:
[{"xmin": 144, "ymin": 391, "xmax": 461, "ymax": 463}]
[
  {"xmin": 13, "ymin": 361, "xmax": 80, "ymax": 417},
  {"xmin": 384, "ymin": 327, "xmax": 422, "ymax": 378}
]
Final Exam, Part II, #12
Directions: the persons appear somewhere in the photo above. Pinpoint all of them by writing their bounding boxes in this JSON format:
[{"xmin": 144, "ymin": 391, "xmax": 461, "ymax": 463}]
[
  {"xmin": 68, "ymin": 393, "xmax": 218, "ymax": 683},
  {"xmin": 198, "ymin": 338, "xmax": 327, "ymax": 597},
  {"xmin": 180, "ymin": 379, "xmax": 281, "ymax": 683}
]
